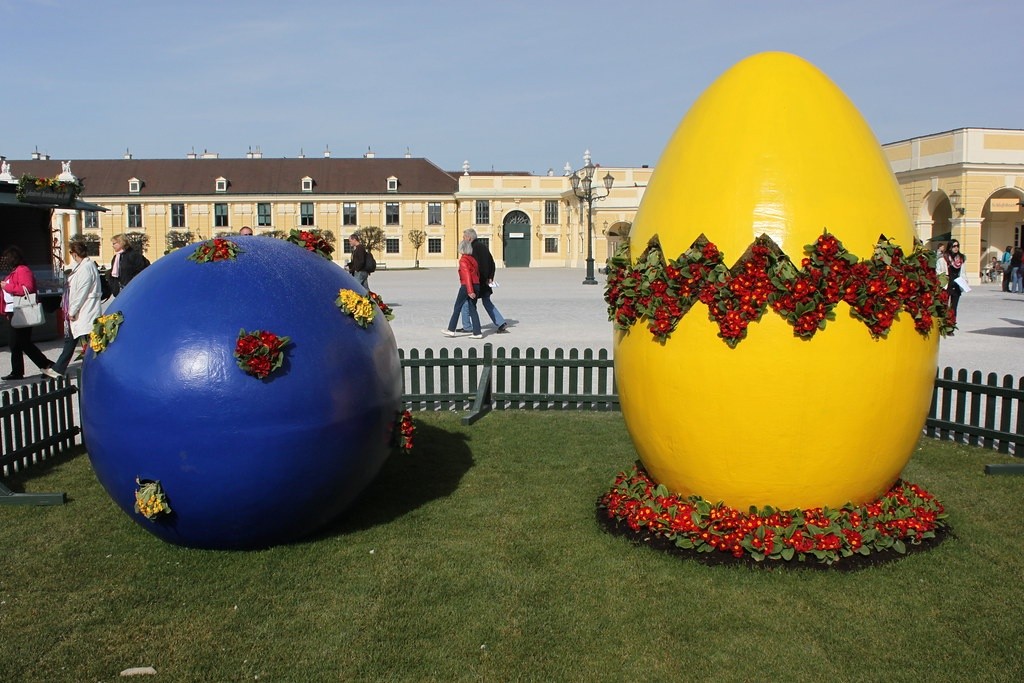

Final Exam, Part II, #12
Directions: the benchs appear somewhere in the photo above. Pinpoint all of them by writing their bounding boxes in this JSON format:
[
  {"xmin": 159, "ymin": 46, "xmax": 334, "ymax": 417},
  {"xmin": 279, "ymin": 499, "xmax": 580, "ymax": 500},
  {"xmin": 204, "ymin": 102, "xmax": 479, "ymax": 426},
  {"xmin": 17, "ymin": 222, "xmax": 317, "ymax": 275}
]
[{"xmin": 375, "ymin": 262, "xmax": 386, "ymax": 271}]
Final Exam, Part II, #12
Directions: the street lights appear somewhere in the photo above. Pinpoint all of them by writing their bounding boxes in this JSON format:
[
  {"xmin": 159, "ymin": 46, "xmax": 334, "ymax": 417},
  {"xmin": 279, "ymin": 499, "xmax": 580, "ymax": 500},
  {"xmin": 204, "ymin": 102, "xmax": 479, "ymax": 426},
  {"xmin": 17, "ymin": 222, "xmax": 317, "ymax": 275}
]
[{"xmin": 568, "ymin": 159, "xmax": 614, "ymax": 285}]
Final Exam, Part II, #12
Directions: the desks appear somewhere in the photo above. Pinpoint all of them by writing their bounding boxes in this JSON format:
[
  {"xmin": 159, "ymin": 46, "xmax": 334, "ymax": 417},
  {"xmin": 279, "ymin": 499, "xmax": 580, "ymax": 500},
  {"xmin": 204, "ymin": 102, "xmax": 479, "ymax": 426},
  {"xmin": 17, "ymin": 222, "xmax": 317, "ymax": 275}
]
[{"xmin": 981, "ymin": 266, "xmax": 993, "ymax": 284}]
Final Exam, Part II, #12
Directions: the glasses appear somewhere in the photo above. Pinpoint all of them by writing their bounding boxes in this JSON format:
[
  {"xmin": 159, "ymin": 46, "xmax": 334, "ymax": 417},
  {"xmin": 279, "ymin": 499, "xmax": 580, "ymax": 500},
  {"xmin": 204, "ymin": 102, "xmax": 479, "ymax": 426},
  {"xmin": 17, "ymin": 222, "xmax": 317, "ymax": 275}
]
[
  {"xmin": 113, "ymin": 242, "xmax": 118, "ymax": 245},
  {"xmin": 952, "ymin": 245, "xmax": 959, "ymax": 248},
  {"xmin": 70, "ymin": 251, "xmax": 76, "ymax": 255}
]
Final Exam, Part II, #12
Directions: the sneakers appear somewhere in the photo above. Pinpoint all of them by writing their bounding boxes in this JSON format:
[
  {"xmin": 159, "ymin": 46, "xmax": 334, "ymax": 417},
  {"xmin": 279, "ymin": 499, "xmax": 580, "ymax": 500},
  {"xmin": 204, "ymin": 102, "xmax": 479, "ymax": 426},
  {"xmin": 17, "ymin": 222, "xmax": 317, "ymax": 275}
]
[{"xmin": 40, "ymin": 367, "xmax": 63, "ymax": 379}]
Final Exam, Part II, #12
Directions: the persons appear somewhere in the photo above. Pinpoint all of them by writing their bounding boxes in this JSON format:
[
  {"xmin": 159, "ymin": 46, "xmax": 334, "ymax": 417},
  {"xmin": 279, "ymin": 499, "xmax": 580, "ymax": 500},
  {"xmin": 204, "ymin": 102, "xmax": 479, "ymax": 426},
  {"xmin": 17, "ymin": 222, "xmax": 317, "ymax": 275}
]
[
  {"xmin": 1001, "ymin": 246, "xmax": 1013, "ymax": 292},
  {"xmin": 936, "ymin": 239, "xmax": 966, "ymax": 316},
  {"xmin": 344, "ymin": 234, "xmax": 368, "ymax": 289},
  {"xmin": 1010, "ymin": 247, "xmax": 1023, "ymax": 293},
  {"xmin": 441, "ymin": 240, "xmax": 483, "ymax": 339},
  {"xmin": 0, "ymin": 246, "xmax": 56, "ymax": 380},
  {"xmin": 990, "ymin": 257, "xmax": 1000, "ymax": 281},
  {"xmin": 240, "ymin": 226, "xmax": 253, "ymax": 236},
  {"xmin": 41, "ymin": 241, "xmax": 103, "ymax": 378},
  {"xmin": 935, "ymin": 243, "xmax": 945, "ymax": 258},
  {"xmin": 456, "ymin": 229, "xmax": 507, "ymax": 333},
  {"xmin": 111, "ymin": 234, "xmax": 144, "ymax": 290}
]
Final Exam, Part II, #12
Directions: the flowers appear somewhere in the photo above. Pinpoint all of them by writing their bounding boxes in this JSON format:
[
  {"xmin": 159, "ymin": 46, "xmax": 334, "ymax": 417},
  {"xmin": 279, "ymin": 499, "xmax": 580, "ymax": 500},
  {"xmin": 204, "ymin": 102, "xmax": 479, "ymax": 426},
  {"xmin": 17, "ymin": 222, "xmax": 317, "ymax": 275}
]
[
  {"xmin": 596, "ymin": 229, "xmax": 958, "ymax": 347},
  {"xmin": 335, "ymin": 287, "xmax": 378, "ymax": 329},
  {"xmin": 368, "ymin": 290, "xmax": 397, "ymax": 323},
  {"xmin": 130, "ymin": 477, "xmax": 171, "ymax": 519},
  {"xmin": 396, "ymin": 409, "xmax": 421, "ymax": 458},
  {"xmin": 89, "ymin": 312, "xmax": 123, "ymax": 352},
  {"xmin": 599, "ymin": 461, "xmax": 951, "ymax": 562},
  {"xmin": 11, "ymin": 170, "xmax": 84, "ymax": 199},
  {"xmin": 287, "ymin": 226, "xmax": 335, "ymax": 262},
  {"xmin": 232, "ymin": 326, "xmax": 290, "ymax": 382},
  {"xmin": 190, "ymin": 236, "xmax": 245, "ymax": 263}
]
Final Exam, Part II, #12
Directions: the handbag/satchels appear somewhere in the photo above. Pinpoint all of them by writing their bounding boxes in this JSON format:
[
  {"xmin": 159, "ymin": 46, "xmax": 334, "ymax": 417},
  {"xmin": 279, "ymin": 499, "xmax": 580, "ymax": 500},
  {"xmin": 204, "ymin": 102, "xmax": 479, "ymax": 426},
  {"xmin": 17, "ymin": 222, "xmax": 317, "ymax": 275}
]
[
  {"xmin": 11, "ymin": 284, "xmax": 46, "ymax": 328},
  {"xmin": 98, "ymin": 271, "xmax": 113, "ymax": 300},
  {"xmin": 1001, "ymin": 261, "xmax": 1008, "ymax": 272}
]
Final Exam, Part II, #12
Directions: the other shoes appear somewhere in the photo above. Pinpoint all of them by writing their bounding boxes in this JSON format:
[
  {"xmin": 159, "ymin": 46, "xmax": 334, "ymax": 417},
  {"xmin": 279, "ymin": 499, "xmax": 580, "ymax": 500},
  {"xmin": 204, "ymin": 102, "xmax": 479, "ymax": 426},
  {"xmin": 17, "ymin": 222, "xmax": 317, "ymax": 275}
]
[
  {"xmin": 1, "ymin": 372, "xmax": 23, "ymax": 380},
  {"xmin": 456, "ymin": 327, "xmax": 473, "ymax": 332},
  {"xmin": 441, "ymin": 329, "xmax": 455, "ymax": 337},
  {"xmin": 497, "ymin": 322, "xmax": 507, "ymax": 333},
  {"xmin": 468, "ymin": 334, "xmax": 482, "ymax": 339},
  {"xmin": 41, "ymin": 361, "xmax": 56, "ymax": 379}
]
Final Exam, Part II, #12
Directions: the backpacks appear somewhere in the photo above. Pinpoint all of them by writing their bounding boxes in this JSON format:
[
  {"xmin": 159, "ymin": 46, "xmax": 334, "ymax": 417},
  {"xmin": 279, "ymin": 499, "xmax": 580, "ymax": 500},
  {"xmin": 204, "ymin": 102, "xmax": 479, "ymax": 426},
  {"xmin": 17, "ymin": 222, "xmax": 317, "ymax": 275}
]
[
  {"xmin": 131, "ymin": 251, "xmax": 151, "ymax": 275},
  {"xmin": 362, "ymin": 252, "xmax": 376, "ymax": 272}
]
